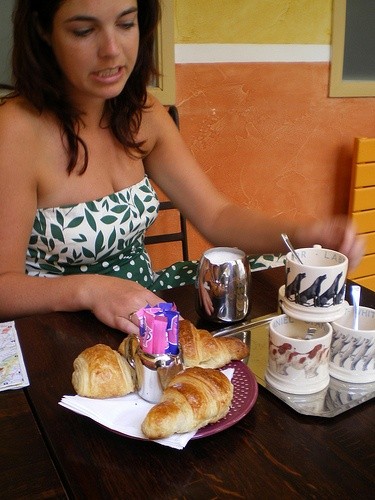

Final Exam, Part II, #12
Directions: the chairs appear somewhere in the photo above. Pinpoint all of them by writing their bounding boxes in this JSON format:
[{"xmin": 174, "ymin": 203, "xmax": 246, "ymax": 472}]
[{"xmin": 142, "ymin": 106, "xmax": 188, "ymax": 272}]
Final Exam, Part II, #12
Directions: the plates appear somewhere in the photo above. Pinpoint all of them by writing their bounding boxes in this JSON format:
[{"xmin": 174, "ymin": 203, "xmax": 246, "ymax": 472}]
[{"xmin": 189, "ymin": 359, "xmax": 258, "ymax": 440}]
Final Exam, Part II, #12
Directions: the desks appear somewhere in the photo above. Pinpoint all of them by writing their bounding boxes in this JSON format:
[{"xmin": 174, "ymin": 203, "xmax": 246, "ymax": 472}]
[{"xmin": 0, "ymin": 265, "xmax": 375, "ymax": 500}]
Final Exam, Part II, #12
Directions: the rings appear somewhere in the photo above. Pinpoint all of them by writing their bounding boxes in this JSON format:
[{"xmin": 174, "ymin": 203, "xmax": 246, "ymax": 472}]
[{"xmin": 128, "ymin": 311, "xmax": 138, "ymax": 320}]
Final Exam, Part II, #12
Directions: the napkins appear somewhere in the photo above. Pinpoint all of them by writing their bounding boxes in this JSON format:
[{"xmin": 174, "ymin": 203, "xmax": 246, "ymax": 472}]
[{"xmin": 58, "ymin": 366, "xmax": 234, "ymax": 450}]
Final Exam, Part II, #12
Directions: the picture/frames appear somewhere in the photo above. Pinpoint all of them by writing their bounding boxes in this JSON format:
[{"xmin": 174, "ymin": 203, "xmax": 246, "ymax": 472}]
[{"xmin": 0, "ymin": 0, "xmax": 177, "ymax": 107}]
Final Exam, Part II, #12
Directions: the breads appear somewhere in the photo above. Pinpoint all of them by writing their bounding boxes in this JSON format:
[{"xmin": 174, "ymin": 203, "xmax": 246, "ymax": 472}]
[
  {"xmin": 141, "ymin": 365, "xmax": 234, "ymax": 438},
  {"xmin": 72, "ymin": 344, "xmax": 136, "ymax": 398},
  {"xmin": 119, "ymin": 319, "xmax": 248, "ymax": 369}
]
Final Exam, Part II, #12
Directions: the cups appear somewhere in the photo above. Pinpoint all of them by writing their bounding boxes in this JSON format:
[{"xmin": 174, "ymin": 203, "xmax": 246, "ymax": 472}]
[
  {"xmin": 278, "ymin": 243, "xmax": 349, "ymax": 323},
  {"xmin": 329, "ymin": 305, "xmax": 375, "ymax": 383},
  {"xmin": 125, "ymin": 334, "xmax": 184, "ymax": 404},
  {"xmin": 265, "ymin": 313, "xmax": 331, "ymax": 395},
  {"xmin": 197, "ymin": 245, "xmax": 251, "ymax": 323}
]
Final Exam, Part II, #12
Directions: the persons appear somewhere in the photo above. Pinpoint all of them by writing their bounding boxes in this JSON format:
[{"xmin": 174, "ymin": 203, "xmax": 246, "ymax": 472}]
[{"xmin": 0, "ymin": 0, "xmax": 365, "ymax": 337}]
